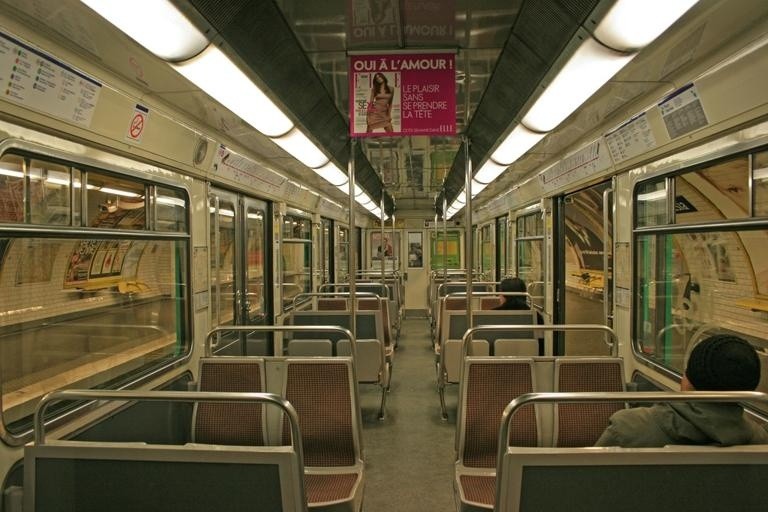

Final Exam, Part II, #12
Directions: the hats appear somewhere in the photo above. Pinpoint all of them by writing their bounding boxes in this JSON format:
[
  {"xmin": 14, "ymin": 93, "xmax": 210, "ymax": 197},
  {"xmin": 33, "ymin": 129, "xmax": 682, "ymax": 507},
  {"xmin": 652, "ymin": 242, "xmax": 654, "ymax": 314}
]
[{"xmin": 686, "ymin": 334, "xmax": 760, "ymax": 391}]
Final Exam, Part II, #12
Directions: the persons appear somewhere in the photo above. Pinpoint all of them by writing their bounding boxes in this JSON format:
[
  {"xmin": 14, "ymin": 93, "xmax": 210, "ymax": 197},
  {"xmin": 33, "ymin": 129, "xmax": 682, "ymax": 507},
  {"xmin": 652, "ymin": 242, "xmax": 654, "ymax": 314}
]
[
  {"xmin": 590, "ymin": 331, "xmax": 767, "ymax": 447},
  {"xmin": 378, "ymin": 238, "xmax": 392, "ymax": 256},
  {"xmin": 365, "ymin": 73, "xmax": 395, "ymax": 133},
  {"xmin": 489, "ymin": 278, "xmax": 546, "ymax": 355}
]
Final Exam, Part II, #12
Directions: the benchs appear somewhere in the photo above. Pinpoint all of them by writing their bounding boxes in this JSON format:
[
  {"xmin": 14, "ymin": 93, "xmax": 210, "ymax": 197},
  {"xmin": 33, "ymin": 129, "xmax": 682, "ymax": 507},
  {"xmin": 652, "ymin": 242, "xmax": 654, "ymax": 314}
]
[
  {"xmin": 429, "ymin": 268, "xmax": 768, "ymax": 512},
  {"xmin": 23, "ymin": 275, "xmax": 404, "ymax": 512}
]
